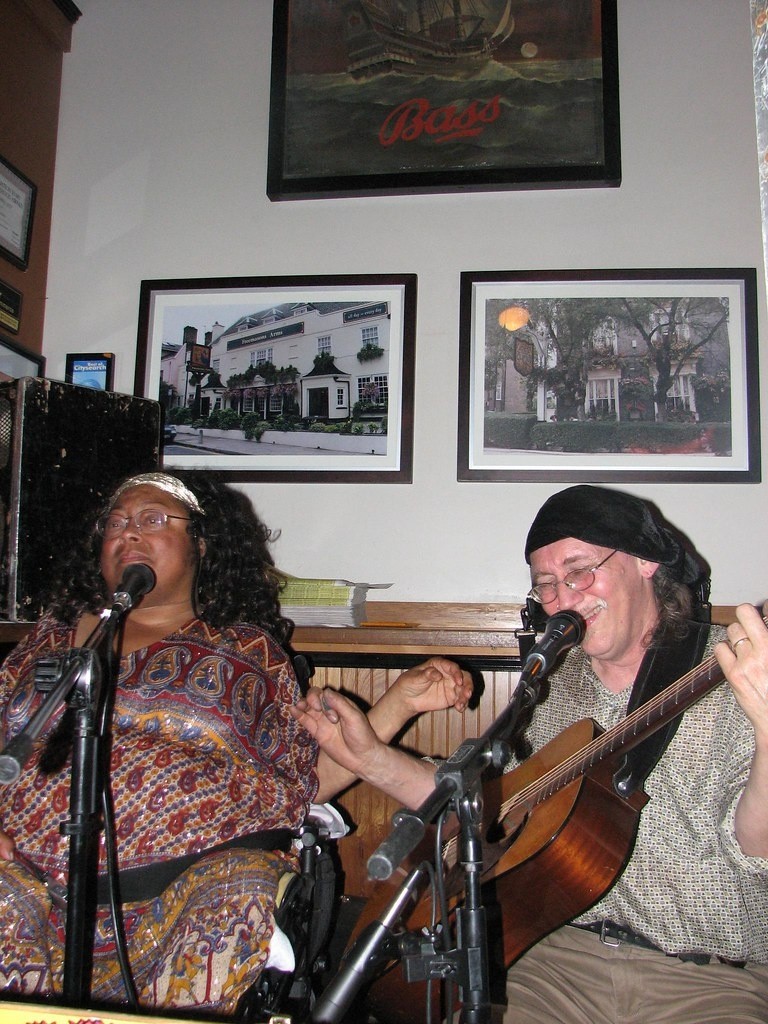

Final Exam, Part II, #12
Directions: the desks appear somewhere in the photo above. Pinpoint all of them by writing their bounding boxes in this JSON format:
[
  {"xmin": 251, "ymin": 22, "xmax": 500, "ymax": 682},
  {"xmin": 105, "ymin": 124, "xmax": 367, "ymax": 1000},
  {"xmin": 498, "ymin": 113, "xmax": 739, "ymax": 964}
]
[{"xmin": 280, "ymin": 603, "xmax": 760, "ymax": 671}]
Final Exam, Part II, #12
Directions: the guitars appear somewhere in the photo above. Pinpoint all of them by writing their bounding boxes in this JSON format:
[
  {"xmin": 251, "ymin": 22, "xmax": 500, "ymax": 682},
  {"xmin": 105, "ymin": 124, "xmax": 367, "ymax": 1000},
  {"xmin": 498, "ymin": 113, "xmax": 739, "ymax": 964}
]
[{"xmin": 340, "ymin": 599, "xmax": 768, "ymax": 1024}]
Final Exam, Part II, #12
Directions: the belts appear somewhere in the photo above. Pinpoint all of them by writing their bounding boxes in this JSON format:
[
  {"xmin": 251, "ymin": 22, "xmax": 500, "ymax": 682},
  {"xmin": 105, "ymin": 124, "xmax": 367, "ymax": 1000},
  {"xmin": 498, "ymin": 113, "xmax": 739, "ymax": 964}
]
[{"xmin": 566, "ymin": 916, "xmax": 668, "ymax": 954}]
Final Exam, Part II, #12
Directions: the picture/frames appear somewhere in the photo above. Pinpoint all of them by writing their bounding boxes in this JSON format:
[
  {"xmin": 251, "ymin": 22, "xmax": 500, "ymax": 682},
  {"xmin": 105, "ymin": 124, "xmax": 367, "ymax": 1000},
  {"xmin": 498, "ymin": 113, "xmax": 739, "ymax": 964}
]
[
  {"xmin": 457, "ymin": 267, "xmax": 762, "ymax": 484},
  {"xmin": 65, "ymin": 353, "xmax": 115, "ymax": 392},
  {"xmin": 135, "ymin": 273, "xmax": 418, "ymax": 484},
  {"xmin": 266, "ymin": 0, "xmax": 622, "ymax": 201}
]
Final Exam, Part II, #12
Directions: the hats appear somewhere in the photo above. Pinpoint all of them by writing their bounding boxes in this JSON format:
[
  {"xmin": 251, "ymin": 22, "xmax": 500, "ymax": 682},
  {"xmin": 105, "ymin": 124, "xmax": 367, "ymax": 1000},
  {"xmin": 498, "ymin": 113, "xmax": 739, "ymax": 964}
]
[{"xmin": 524, "ymin": 483, "xmax": 711, "ymax": 591}]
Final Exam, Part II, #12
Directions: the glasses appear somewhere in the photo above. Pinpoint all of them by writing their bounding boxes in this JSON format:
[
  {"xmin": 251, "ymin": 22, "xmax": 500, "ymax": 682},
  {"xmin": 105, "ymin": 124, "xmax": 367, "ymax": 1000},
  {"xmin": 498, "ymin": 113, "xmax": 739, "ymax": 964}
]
[
  {"xmin": 95, "ymin": 510, "xmax": 194, "ymax": 540},
  {"xmin": 526, "ymin": 550, "xmax": 617, "ymax": 604}
]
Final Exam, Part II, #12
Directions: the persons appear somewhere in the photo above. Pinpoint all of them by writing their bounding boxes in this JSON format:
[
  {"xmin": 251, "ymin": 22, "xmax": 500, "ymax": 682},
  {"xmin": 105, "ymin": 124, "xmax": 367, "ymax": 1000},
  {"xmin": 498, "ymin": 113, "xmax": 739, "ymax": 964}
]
[
  {"xmin": 0, "ymin": 473, "xmax": 473, "ymax": 1016},
  {"xmin": 289, "ymin": 485, "xmax": 768, "ymax": 1024}
]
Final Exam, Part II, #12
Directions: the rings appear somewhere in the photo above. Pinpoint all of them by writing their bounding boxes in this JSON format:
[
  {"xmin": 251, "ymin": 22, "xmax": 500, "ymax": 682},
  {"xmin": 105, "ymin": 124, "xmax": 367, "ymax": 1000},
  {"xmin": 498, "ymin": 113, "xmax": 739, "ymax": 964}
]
[{"xmin": 733, "ymin": 638, "xmax": 750, "ymax": 652}]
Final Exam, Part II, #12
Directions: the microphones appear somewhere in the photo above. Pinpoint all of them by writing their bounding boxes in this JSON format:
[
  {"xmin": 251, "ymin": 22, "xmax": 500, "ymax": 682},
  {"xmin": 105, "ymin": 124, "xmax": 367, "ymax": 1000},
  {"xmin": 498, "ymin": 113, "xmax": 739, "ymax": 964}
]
[
  {"xmin": 519, "ymin": 608, "xmax": 587, "ymax": 690},
  {"xmin": 110, "ymin": 563, "xmax": 155, "ymax": 620},
  {"xmin": 310, "ymin": 869, "xmax": 423, "ymax": 1024}
]
